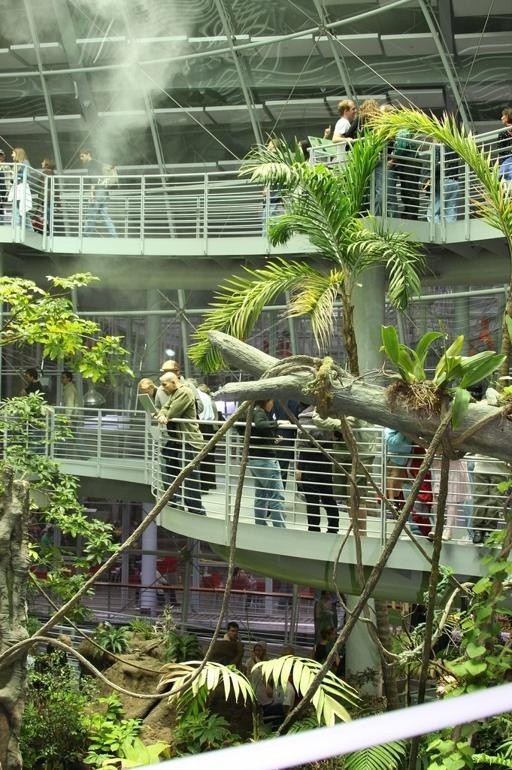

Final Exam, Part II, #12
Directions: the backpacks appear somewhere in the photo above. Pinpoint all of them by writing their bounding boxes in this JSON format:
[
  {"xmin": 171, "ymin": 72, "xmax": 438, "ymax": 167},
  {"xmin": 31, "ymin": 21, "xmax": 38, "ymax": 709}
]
[{"xmin": 90, "ymin": 158, "xmax": 119, "ymax": 190}]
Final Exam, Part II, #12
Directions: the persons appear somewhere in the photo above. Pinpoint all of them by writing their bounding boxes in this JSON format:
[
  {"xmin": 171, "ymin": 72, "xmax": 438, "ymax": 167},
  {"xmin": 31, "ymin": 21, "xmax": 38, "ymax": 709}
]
[
  {"xmin": 213, "ymin": 622, "xmax": 245, "ymax": 668},
  {"xmin": 259, "ymin": 99, "xmax": 512, "ymax": 224},
  {"xmin": 0, "ymin": 150, "xmax": 10, "ymax": 225},
  {"xmin": 138, "ymin": 360, "xmax": 225, "ymax": 516},
  {"xmin": 57, "ymin": 371, "xmax": 91, "ymax": 460},
  {"xmin": 77, "ymin": 146, "xmax": 118, "ymax": 238},
  {"xmin": 7, "ymin": 147, "xmax": 34, "ymax": 231},
  {"xmin": 233, "ymin": 399, "xmax": 432, "ymax": 538},
  {"xmin": 18, "ymin": 369, "xmax": 44, "ymax": 455},
  {"xmin": 428, "ymin": 452, "xmax": 511, "ymax": 543},
  {"xmin": 28, "ymin": 517, "xmax": 183, "ymax": 609},
  {"xmin": 31, "ymin": 158, "xmax": 65, "ymax": 236},
  {"xmin": 244, "ymin": 588, "xmax": 347, "ymax": 717}
]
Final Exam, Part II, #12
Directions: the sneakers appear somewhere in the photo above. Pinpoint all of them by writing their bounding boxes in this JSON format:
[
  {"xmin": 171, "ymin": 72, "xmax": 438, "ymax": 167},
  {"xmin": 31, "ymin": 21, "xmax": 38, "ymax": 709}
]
[{"xmin": 429, "ymin": 526, "xmax": 492, "ymax": 544}]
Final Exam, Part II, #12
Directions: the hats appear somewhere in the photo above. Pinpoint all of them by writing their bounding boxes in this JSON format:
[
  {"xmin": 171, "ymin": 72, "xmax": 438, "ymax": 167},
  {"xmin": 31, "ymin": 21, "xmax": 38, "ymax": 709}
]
[{"xmin": 159, "ymin": 360, "xmax": 179, "ymax": 373}]
